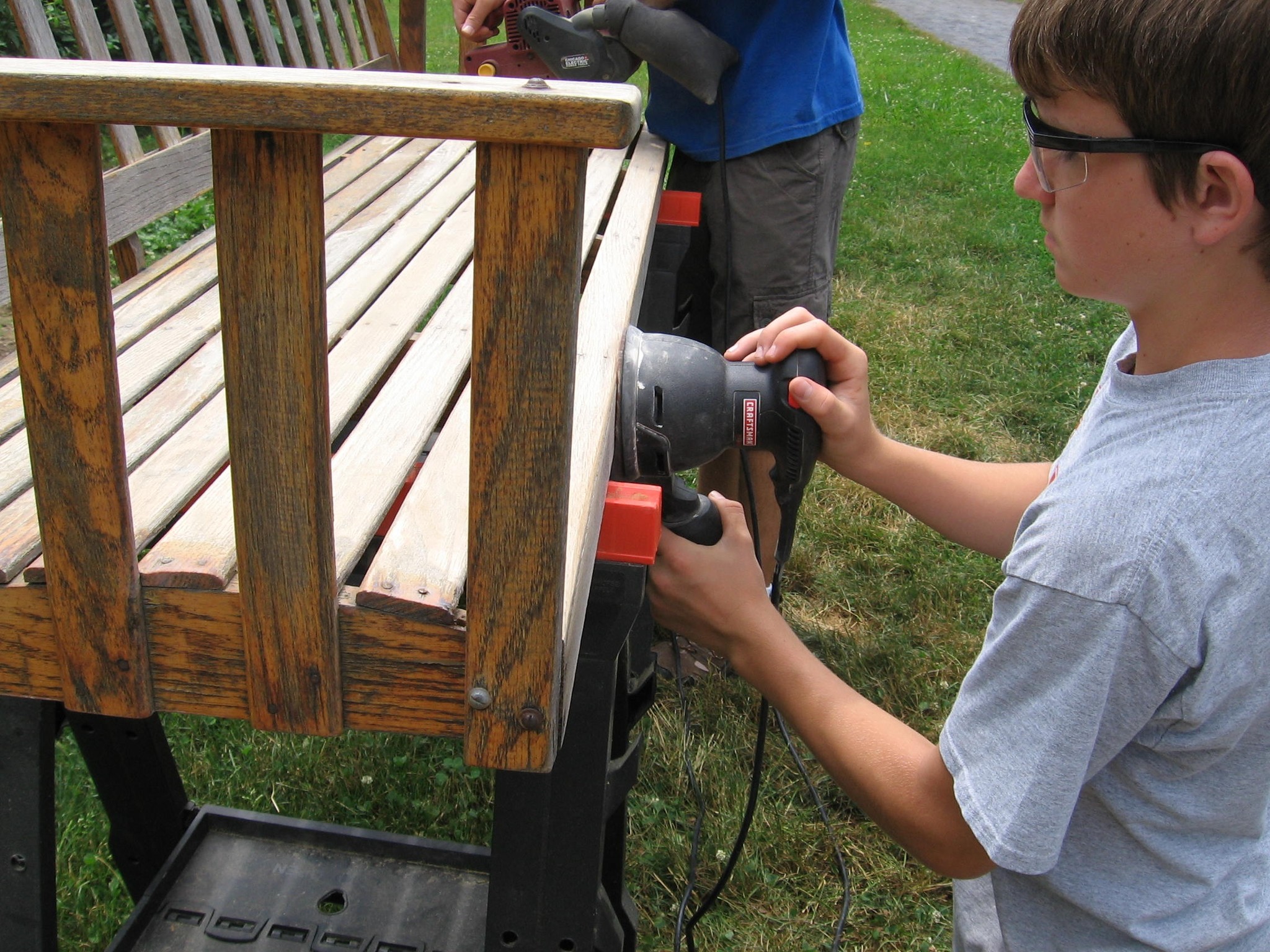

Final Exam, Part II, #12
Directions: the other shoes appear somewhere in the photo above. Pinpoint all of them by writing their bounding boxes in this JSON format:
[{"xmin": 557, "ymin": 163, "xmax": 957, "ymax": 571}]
[{"xmin": 650, "ymin": 626, "xmax": 735, "ymax": 688}]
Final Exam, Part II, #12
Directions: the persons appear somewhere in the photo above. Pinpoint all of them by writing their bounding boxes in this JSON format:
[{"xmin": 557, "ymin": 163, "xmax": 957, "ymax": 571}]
[
  {"xmin": 450, "ymin": 1, "xmax": 864, "ymax": 686},
  {"xmin": 642, "ymin": 1, "xmax": 1270, "ymax": 951}
]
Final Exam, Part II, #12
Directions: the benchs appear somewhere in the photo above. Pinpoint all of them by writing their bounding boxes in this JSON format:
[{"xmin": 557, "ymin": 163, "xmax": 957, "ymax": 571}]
[{"xmin": 3, "ymin": 0, "xmax": 673, "ymax": 952}]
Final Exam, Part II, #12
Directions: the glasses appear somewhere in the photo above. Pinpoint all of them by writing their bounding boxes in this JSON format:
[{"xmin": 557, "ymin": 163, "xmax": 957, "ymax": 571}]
[{"xmin": 1020, "ymin": 93, "xmax": 1270, "ymax": 212}]
